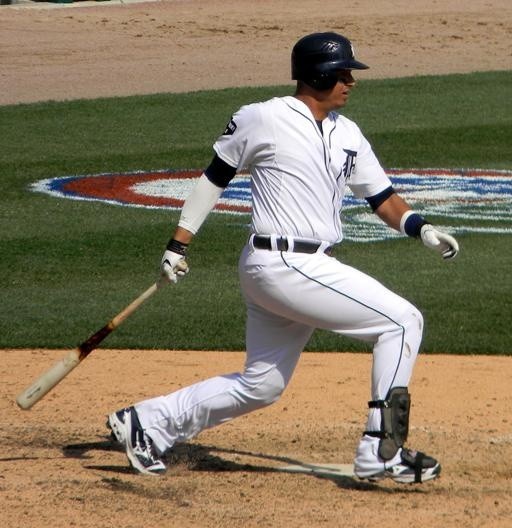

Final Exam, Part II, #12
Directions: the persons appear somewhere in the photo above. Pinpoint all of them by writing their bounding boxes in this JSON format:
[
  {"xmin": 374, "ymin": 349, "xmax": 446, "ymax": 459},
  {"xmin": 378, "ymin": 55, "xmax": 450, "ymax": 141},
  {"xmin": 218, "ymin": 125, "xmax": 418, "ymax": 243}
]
[{"xmin": 104, "ymin": 29, "xmax": 461, "ymax": 483}]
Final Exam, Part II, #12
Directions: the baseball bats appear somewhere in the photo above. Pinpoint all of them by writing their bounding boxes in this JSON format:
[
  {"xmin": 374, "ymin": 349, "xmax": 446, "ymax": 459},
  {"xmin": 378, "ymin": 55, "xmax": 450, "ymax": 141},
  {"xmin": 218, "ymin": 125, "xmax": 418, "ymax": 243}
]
[{"xmin": 16, "ymin": 258, "xmax": 188, "ymax": 410}]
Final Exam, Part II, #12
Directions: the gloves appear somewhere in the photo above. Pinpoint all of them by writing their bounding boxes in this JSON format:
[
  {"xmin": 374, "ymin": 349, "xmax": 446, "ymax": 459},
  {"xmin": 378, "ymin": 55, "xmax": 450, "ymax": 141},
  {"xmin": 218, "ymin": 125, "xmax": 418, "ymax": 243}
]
[
  {"xmin": 398, "ymin": 208, "xmax": 461, "ymax": 261},
  {"xmin": 158, "ymin": 236, "xmax": 192, "ymax": 285}
]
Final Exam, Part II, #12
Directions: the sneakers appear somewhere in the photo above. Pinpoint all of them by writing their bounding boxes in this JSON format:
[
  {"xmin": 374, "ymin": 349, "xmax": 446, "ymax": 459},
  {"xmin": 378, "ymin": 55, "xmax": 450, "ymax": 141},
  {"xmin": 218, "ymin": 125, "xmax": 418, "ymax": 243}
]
[
  {"xmin": 355, "ymin": 445, "xmax": 442, "ymax": 484},
  {"xmin": 105, "ymin": 404, "xmax": 168, "ymax": 477}
]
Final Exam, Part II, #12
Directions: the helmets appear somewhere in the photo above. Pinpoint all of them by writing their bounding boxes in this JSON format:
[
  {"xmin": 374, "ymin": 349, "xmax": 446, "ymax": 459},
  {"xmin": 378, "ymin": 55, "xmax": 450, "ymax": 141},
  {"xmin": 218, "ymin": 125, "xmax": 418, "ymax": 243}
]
[{"xmin": 289, "ymin": 30, "xmax": 372, "ymax": 81}]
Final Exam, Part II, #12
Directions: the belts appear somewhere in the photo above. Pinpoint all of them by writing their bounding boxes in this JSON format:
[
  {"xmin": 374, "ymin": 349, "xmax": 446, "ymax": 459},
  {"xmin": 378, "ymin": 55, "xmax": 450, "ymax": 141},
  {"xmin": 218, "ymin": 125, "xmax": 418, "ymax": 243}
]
[{"xmin": 247, "ymin": 231, "xmax": 334, "ymax": 256}]
